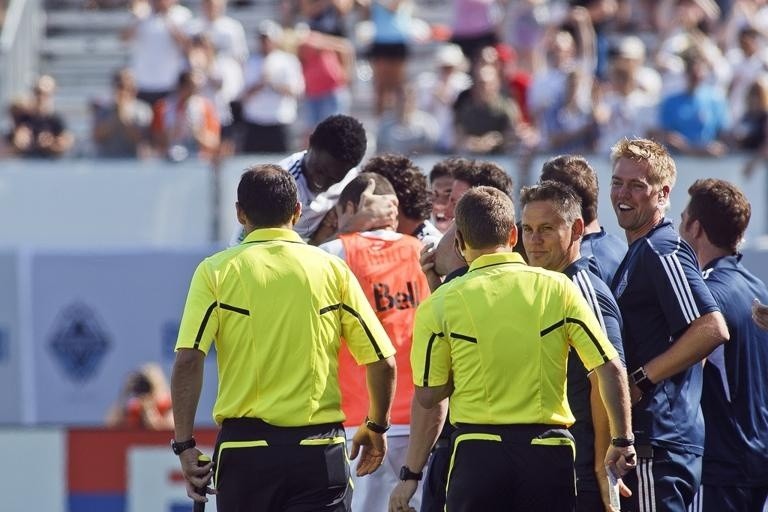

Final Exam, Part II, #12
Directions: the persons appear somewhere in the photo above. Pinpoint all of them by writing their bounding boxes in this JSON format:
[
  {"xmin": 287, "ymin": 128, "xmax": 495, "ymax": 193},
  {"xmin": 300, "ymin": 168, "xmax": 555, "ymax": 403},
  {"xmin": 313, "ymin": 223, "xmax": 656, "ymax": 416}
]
[
  {"xmin": 610, "ymin": 132, "xmax": 731, "ymax": 512},
  {"xmin": 231, "ymin": 114, "xmax": 364, "ymax": 252},
  {"xmin": 747, "ymin": 293, "xmax": 767, "ymax": 332},
  {"xmin": 678, "ymin": 175, "xmax": 767, "ymax": 511},
  {"xmin": 171, "ymin": 164, "xmax": 397, "ymax": 511},
  {"xmin": 105, "ymin": 361, "xmax": 179, "ymax": 431}
]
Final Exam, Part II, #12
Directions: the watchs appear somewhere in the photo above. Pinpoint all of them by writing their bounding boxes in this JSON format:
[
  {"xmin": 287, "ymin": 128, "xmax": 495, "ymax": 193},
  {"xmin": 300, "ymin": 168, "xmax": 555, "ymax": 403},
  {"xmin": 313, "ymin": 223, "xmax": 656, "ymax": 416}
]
[{"xmin": 631, "ymin": 366, "xmax": 653, "ymax": 393}]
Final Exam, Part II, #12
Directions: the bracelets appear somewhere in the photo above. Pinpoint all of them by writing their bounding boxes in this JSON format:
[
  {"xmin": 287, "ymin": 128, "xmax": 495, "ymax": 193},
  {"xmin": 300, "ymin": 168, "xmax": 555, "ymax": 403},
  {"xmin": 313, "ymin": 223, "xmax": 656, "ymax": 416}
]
[
  {"xmin": 363, "ymin": 415, "xmax": 391, "ymax": 435},
  {"xmin": 171, "ymin": 437, "xmax": 195, "ymax": 457}
]
